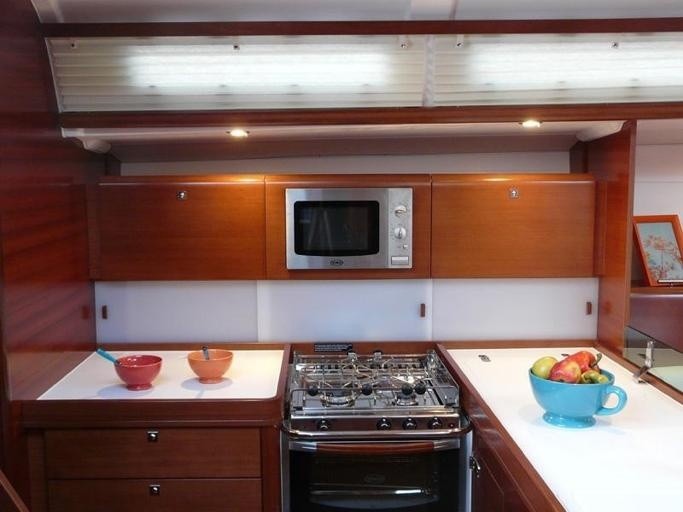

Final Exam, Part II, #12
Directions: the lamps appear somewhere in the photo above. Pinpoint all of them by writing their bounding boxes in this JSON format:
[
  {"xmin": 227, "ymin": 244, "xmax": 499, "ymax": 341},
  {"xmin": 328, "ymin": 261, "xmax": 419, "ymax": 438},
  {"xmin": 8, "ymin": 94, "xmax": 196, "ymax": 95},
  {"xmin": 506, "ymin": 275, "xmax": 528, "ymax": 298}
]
[{"xmin": 43, "ymin": 31, "xmax": 683, "ymax": 115}]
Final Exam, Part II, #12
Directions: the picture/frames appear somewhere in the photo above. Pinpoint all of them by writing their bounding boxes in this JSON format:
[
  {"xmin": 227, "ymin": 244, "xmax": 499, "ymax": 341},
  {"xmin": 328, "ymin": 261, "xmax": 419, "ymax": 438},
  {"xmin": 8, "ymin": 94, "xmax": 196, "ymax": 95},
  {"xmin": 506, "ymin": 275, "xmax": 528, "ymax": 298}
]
[{"xmin": 632, "ymin": 215, "xmax": 683, "ymax": 287}]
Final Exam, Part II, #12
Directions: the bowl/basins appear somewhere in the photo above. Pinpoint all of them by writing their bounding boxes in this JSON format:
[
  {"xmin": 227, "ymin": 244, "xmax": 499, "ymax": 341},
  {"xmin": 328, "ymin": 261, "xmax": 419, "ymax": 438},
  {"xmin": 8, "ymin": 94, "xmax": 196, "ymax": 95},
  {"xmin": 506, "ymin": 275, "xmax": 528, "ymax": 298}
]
[
  {"xmin": 114, "ymin": 355, "xmax": 162, "ymax": 392},
  {"xmin": 527, "ymin": 365, "xmax": 627, "ymax": 429},
  {"xmin": 187, "ymin": 350, "xmax": 232, "ymax": 385}
]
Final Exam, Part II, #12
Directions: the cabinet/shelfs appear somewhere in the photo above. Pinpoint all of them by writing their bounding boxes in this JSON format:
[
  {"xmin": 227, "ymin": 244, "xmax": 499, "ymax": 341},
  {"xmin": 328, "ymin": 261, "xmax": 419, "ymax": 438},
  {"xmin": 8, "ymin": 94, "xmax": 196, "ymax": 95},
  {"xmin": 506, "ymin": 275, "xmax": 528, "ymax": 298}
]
[
  {"xmin": 99, "ymin": 173, "xmax": 265, "ymax": 281},
  {"xmin": 431, "ymin": 173, "xmax": 596, "ymax": 279},
  {"xmin": 468, "ymin": 421, "xmax": 566, "ymax": 512},
  {"xmin": 27, "ymin": 400, "xmax": 282, "ymax": 512}
]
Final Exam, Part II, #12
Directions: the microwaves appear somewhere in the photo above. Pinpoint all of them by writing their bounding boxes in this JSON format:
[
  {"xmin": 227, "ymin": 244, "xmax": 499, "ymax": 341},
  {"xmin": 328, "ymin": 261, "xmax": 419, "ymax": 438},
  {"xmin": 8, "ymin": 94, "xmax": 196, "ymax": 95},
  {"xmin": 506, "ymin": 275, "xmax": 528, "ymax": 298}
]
[{"xmin": 282, "ymin": 187, "xmax": 414, "ymax": 270}]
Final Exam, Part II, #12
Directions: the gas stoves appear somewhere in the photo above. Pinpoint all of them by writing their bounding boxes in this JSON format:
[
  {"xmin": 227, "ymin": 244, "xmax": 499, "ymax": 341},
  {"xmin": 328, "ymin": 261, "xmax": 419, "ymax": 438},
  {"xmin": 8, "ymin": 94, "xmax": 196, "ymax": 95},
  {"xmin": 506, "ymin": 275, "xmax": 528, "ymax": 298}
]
[{"xmin": 287, "ymin": 351, "xmax": 462, "ymax": 417}]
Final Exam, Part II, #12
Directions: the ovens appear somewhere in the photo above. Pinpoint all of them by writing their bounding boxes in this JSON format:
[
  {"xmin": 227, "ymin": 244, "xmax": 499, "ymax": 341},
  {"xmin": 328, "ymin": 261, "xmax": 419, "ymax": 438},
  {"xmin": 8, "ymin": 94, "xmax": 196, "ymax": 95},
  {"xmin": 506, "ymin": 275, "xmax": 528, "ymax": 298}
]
[{"xmin": 279, "ymin": 430, "xmax": 473, "ymax": 512}]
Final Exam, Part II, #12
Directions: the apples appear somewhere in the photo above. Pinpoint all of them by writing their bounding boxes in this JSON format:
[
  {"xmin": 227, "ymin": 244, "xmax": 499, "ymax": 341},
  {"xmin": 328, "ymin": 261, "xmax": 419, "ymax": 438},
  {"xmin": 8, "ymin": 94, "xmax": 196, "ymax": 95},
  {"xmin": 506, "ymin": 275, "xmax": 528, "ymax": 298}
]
[{"xmin": 531, "ymin": 350, "xmax": 609, "ymax": 385}]
